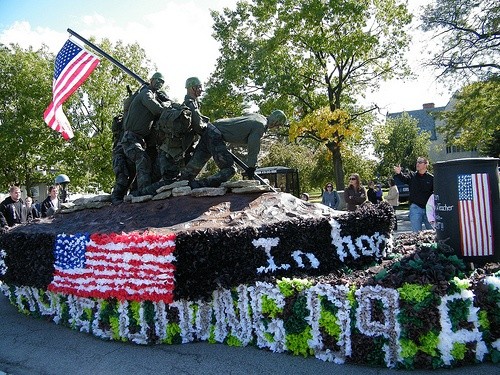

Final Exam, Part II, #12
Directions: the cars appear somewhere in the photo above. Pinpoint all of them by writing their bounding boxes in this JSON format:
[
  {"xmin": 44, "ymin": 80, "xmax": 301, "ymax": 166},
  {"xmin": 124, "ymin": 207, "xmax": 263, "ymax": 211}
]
[{"xmin": 392, "ymin": 176, "xmax": 413, "ymax": 201}]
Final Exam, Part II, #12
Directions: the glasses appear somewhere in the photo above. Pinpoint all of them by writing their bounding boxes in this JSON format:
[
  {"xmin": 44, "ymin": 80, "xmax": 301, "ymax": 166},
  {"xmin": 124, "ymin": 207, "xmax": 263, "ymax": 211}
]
[
  {"xmin": 327, "ymin": 186, "xmax": 332, "ymax": 188},
  {"xmin": 349, "ymin": 178, "xmax": 355, "ymax": 181},
  {"xmin": 415, "ymin": 161, "xmax": 424, "ymax": 164}
]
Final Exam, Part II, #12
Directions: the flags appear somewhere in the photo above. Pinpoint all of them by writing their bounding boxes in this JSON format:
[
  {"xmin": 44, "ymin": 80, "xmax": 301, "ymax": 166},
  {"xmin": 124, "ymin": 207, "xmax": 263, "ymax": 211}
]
[{"xmin": 43, "ymin": 39, "xmax": 101, "ymax": 141}]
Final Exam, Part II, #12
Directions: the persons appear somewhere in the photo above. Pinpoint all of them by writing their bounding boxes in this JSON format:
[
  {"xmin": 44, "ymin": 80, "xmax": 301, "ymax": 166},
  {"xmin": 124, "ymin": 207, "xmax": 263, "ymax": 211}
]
[
  {"xmin": 120, "ymin": 72, "xmax": 210, "ymax": 197},
  {"xmin": 394, "ymin": 156, "xmax": 434, "ymax": 233},
  {"xmin": 174, "ymin": 109, "xmax": 288, "ymax": 189},
  {"xmin": 344, "ymin": 172, "xmax": 367, "ymax": 212},
  {"xmin": 367, "ymin": 180, "xmax": 381, "ymax": 204},
  {"xmin": 384, "ymin": 179, "xmax": 400, "ymax": 230},
  {"xmin": 0, "ymin": 186, "xmax": 27, "ymax": 233},
  {"xmin": 321, "ymin": 183, "xmax": 341, "ymax": 210},
  {"xmin": 18, "ymin": 194, "xmax": 40, "ymax": 221},
  {"xmin": 300, "ymin": 192, "xmax": 310, "ymax": 203},
  {"xmin": 111, "ymin": 94, "xmax": 150, "ymax": 206},
  {"xmin": 374, "ymin": 182, "xmax": 383, "ymax": 204},
  {"xmin": 41, "ymin": 185, "xmax": 63, "ymax": 218}
]
